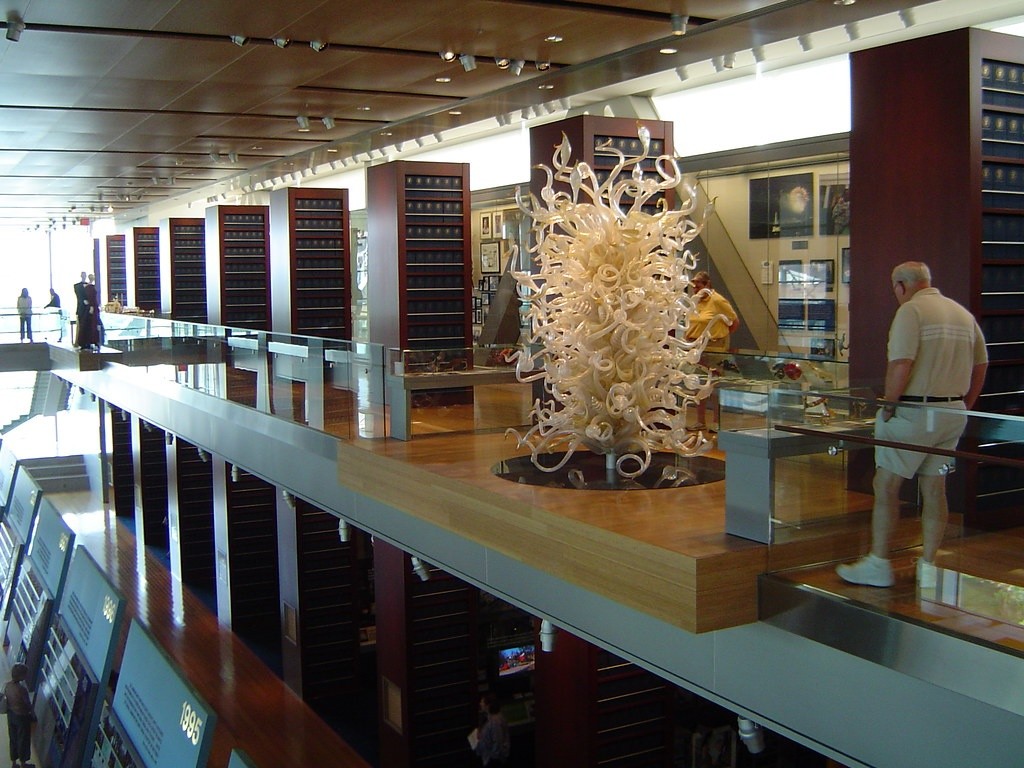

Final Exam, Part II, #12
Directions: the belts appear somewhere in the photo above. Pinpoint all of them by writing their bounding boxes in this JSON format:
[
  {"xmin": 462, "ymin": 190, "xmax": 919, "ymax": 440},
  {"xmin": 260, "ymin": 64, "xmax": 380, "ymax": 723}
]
[{"xmin": 898, "ymin": 395, "xmax": 962, "ymax": 402}]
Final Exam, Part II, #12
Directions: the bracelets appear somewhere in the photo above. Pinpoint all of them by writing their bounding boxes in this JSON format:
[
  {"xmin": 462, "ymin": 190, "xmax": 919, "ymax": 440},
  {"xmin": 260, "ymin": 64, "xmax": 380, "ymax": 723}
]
[{"xmin": 883, "ymin": 404, "xmax": 895, "ymax": 412}]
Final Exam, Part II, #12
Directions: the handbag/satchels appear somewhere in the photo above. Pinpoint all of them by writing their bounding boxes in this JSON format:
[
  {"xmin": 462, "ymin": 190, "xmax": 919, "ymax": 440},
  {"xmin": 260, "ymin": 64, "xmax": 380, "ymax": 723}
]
[{"xmin": 0, "ymin": 682, "xmax": 8, "ymax": 714}]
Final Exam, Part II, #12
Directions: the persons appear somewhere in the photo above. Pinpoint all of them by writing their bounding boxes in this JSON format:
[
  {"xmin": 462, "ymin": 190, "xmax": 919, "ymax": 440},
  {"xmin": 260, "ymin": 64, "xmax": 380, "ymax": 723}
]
[
  {"xmin": 696, "ymin": 742, "xmax": 714, "ymax": 767},
  {"xmin": 683, "ymin": 270, "xmax": 740, "ymax": 434},
  {"xmin": 714, "ymin": 740, "xmax": 731, "ymax": 768},
  {"xmin": 73, "ymin": 272, "xmax": 106, "ymax": 351},
  {"xmin": 44, "ymin": 289, "xmax": 64, "ymax": 342},
  {"xmin": 832, "ymin": 264, "xmax": 989, "ymax": 588},
  {"xmin": 477, "ymin": 695, "xmax": 511, "ymax": 768},
  {"xmin": 0, "ymin": 663, "xmax": 38, "ymax": 768},
  {"xmin": 16, "ymin": 288, "xmax": 34, "ymax": 344}
]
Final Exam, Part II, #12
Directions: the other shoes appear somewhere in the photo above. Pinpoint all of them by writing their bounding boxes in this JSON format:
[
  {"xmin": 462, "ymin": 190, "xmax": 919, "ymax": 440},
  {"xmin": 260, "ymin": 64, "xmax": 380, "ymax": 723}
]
[
  {"xmin": 12, "ymin": 764, "xmax": 20, "ymax": 768},
  {"xmin": 21, "ymin": 764, "xmax": 35, "ymax": 768}
]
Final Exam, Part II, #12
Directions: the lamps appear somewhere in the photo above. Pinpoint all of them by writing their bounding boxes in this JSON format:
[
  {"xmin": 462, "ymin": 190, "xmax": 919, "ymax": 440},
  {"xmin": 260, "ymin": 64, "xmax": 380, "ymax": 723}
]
[
  {"xmin": 282, "ymin": 489, "xmax": 295, "ymax": 510},
  {"xmin": 539, "ymin": 619, "xmax": 557, "ymax": 652},
  {"xmin": 231, "ymin": 464, "xmax": 241, "ymax": 482},
  {"xmin": 197, "ymin": 447, "xmax": 207, "ymax": 462},
  {"xmin": 310, "ymin": 39, "xmax": 329, "ymax": 52},
  {"xmin": 274, "ymin": 37, "xmax": 291, "ymax": 48},
  {"xmin": 411, "ymin": 556, "xmax": 430, "ymax": 581},
  {"xmin": 6, "ymin": 18, "xmax": 25, "ymax": 42},
  {"xmin": 57, "ymin": 376, "xmax": 173, "ymax": 444},
  {"xmin": 670, "ymin": 0, "xmax": 917, "ymax": 82},
  {"xmin": 738, "ymin": 715, "xmax": 765, "ymax": 753},
  {"xmin": 27, "ymin": 116, "xmax": 444, "ymax": 233},
  {"xmin": 494, "ymin": 95, "xmax": 571, "ymax": 128},
  {"xmin": 337, "ymin": 518, "xmax": 351, "ymax": 543},
  {"xmin": 440, "ymin": 49, "xmax": 551, "ymax": 75},
  {"xmin": 230, "ymin": 34, "xmax": 248, "ymax": 45}
]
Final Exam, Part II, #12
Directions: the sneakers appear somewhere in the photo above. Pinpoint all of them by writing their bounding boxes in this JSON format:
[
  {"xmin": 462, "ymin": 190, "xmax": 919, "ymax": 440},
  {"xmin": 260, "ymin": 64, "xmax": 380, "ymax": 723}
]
[
  {"xmin": 912, "ymin": 555, "xmax": 937, "ymax": 588},
  {"xmin": 836, "ymin": 556, "xmax": 894, "ymax": 587},
  {"xmin": 686, "ymin": 423, "xmax": 706, "ymax": 431},
  {"xmin": 708, "ymin": 423, "xmax": 719, "ymax": 432}
]
